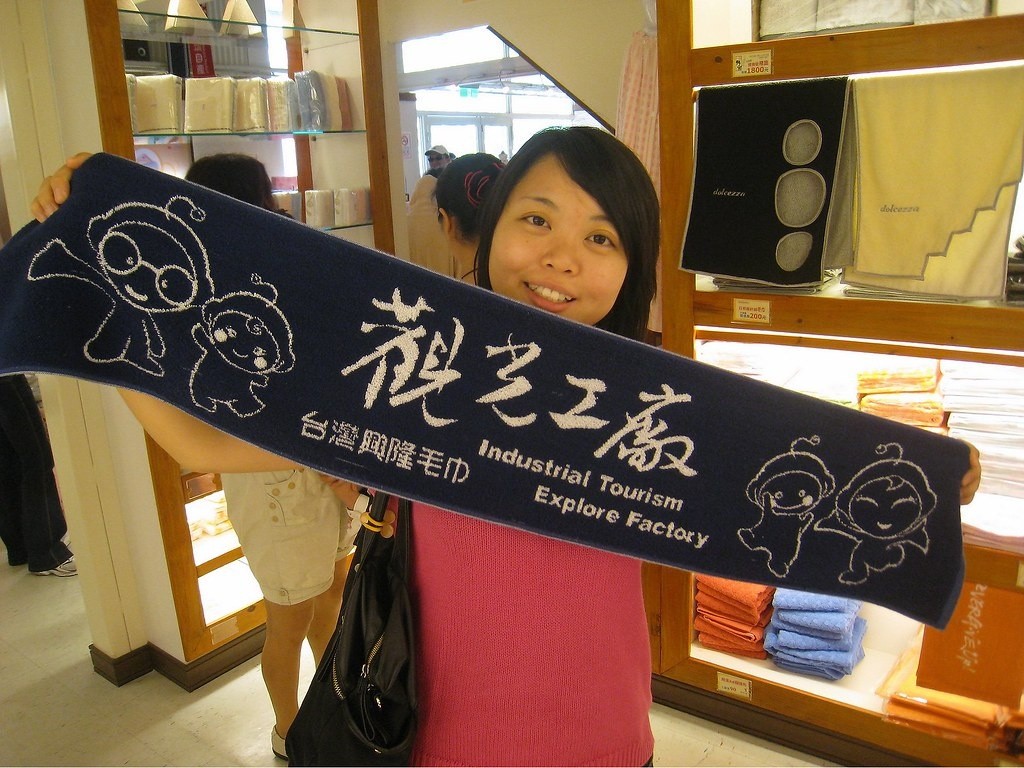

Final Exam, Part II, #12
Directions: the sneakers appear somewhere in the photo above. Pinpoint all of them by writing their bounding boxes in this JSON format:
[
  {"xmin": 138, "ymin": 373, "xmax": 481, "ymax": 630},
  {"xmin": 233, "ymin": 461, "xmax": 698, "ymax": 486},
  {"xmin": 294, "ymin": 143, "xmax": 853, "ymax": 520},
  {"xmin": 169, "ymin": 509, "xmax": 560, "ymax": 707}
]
[
  {"xmin": 33, "ymin": 556, "xmax": 77, "ymax": 577},
  {"xmin": 59, "ymin": 531, "xmax": 71, "ymax": 547}
]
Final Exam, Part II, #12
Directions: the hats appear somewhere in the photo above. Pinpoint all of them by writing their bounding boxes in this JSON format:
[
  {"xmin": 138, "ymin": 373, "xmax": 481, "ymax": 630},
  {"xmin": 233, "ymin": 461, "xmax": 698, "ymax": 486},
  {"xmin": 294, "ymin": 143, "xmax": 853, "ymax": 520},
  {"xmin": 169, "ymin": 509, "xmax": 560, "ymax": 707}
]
[{"xmin": 425, "ymin": 145, "xmax": 449, "ymax": 156}]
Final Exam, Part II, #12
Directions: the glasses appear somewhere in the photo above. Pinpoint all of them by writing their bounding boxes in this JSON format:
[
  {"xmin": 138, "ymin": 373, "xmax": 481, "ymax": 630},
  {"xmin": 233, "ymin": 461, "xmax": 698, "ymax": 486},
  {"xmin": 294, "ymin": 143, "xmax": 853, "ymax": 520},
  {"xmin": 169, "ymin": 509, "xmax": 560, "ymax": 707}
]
[{"xmin": 428, "ymin": 154, "xmax": 447, "ymax": 162}]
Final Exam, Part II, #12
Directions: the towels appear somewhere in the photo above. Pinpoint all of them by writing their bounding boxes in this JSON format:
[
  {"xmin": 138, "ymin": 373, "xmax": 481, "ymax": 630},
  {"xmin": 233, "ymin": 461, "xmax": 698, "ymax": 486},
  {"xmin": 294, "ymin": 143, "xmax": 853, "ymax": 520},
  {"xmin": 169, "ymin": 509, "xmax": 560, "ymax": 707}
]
[
  {"xmin": 685, "ymin": 335, "xmax": 1024, "ymax": 684},
  {"xmin": 0, "ymin": 144, "xmax": 972, "ymax": 634},
  {"xmin": 676, "ymin": 60, "xmax": 1024, "ymax": 313}
]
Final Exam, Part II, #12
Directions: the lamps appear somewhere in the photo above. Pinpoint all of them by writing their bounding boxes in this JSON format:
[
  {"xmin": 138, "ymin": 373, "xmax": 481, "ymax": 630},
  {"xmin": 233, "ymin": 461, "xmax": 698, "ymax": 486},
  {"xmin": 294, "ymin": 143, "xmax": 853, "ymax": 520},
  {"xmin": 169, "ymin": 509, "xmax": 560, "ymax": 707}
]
[
  {"xmin": 164, "ymin": 0, "xmax": 214, "ymax": 34},
  {"xmin": 116, "ymin": 0, "xmax": 149, "ymax": 27},
  {"xmin": 218, "ymin": 0, "xmax": 264, "ymax": 39}
]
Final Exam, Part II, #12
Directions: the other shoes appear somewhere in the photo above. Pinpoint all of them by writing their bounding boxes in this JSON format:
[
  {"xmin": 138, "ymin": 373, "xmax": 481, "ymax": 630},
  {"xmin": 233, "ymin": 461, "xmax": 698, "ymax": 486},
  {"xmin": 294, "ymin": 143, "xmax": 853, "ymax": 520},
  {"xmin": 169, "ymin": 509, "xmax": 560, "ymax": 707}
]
[{"xmin": 271, "ymin": 725, "xmax": 289, "ymax": 761}]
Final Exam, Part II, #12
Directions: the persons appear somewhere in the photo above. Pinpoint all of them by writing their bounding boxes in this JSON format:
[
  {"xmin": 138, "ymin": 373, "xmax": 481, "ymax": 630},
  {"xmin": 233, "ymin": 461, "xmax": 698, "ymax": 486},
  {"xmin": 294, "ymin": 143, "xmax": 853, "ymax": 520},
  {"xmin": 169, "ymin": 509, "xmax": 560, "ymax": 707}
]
[
  {"xmin": 0, "ymin": 371, "xmax": 77, "ymax": 576},
  {"xmin": 30, "ymin": 124, "xmax": 983, "ymax": 768}
]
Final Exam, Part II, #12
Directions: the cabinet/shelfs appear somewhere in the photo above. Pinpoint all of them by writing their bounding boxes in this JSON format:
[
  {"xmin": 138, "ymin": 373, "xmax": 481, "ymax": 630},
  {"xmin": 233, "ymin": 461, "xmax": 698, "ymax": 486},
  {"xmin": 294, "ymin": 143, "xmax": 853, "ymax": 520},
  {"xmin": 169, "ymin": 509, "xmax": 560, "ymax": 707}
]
[
  {"xmin": 84, "ymin": 0, "xmax": 398, "ymax": 686},
  {"xmin": 654, "ymin": 1, "xmax": 1024, "ymax": 767}
]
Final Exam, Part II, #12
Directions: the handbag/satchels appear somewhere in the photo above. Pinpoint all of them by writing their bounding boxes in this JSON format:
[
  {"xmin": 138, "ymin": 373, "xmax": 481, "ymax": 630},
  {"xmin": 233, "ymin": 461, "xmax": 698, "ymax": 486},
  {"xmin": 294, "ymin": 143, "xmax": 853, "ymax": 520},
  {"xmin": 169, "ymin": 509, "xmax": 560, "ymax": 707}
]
[{"xmin": 284, "ymin": 522, "xmax": 419, "ymax": 767}]
